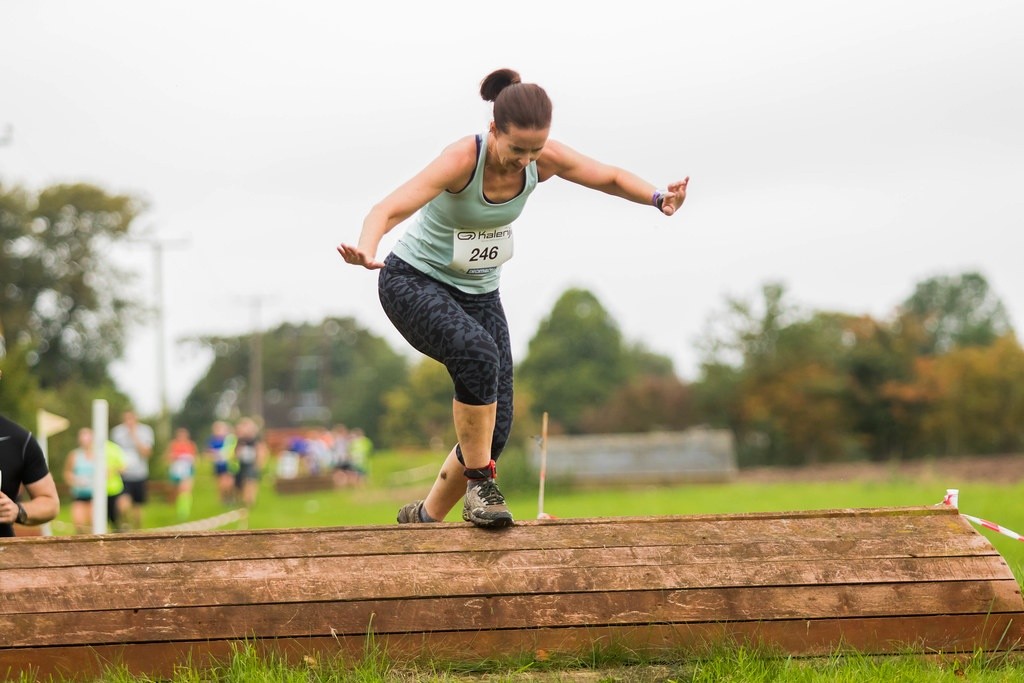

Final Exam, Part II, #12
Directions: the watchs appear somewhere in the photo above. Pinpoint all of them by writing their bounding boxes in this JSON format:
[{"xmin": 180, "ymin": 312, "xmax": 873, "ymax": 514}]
[{"xmin": 15, "ymin": 501, "xmax": 28, "ymax": 524}]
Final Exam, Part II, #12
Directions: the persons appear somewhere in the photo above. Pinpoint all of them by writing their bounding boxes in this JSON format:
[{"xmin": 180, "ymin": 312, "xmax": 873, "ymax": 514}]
[
  {"xmin": 64, "ymin": 410, "xmax": 373, "ymax": 534},
  {"xmin": 337, "ymin": 70, "xmax": 689, "ymax": 528},
  {"xmin": 0, "ymin": 415, "xmax": 61, "ymax": 537}
]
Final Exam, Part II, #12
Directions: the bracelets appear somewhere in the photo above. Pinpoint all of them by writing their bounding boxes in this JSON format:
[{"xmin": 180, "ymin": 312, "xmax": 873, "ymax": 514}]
[{"xmin": 652, "ymin": 190, "xmax": 663, "ymax": 209}]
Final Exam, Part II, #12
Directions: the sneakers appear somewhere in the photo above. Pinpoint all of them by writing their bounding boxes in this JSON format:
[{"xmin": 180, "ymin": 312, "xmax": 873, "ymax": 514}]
[
  {"xmin": 396, "ymin": 500, "xmax": 423, "ymax": 523},
  {"xmin": 462, "ymin": 478, "xmax": 512, "ymax": 530}
]
